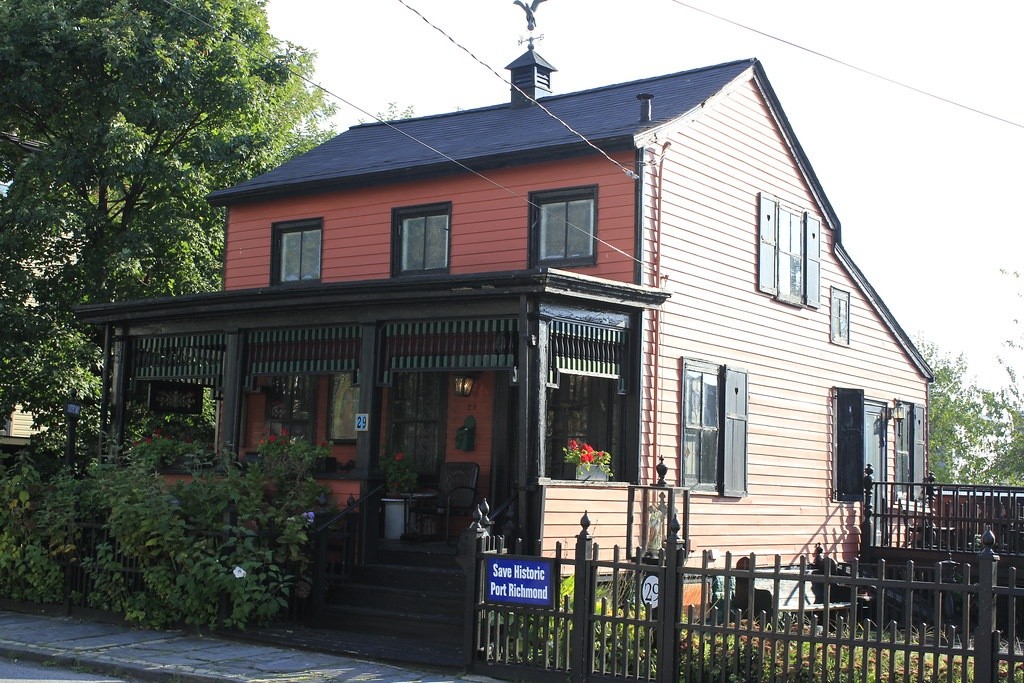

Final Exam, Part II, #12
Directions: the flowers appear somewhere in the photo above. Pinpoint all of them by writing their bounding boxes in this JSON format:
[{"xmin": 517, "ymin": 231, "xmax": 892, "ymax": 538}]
[
  {"xmin": 377, "ymin": 446, "xmax": 418, "ymax": 492},
  {"xmin": 562, "ymin": 439, "xmax": 614, "ymax": 478}
]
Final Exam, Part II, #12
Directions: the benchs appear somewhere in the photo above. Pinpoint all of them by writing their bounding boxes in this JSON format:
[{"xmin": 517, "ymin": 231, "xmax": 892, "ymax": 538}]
[
  {"xmin": 731, "ymin": 555, "xmax": 852, "ymax": 633},
  {"xmin": 900, "ymin": 499, "xmax": 955, "ymax": 549}
]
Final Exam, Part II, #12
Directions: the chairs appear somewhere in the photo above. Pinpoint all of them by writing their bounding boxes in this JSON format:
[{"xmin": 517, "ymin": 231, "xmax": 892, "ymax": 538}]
[{"xmin": 407, "ymin": 461, "xmax": 480, "ymax": 548}]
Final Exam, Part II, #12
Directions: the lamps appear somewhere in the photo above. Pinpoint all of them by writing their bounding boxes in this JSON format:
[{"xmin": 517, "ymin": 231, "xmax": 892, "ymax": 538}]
[
  {"xmin": 888, "ymin": 395, "xmax": 905, "ymax": 438},
  {"xmin": 453, "ymin": 374, "xmax": 474, "ymax": 398}
]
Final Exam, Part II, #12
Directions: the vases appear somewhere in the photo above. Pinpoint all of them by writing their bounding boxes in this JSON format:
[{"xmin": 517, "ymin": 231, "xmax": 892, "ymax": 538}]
[
  {"xmin": 576, "ymin": 462, "xmax": 610, "ymax": 481},
  {"xmin": 389, "ymin": 482, "xmax": 401, "ymax": 499}
]
